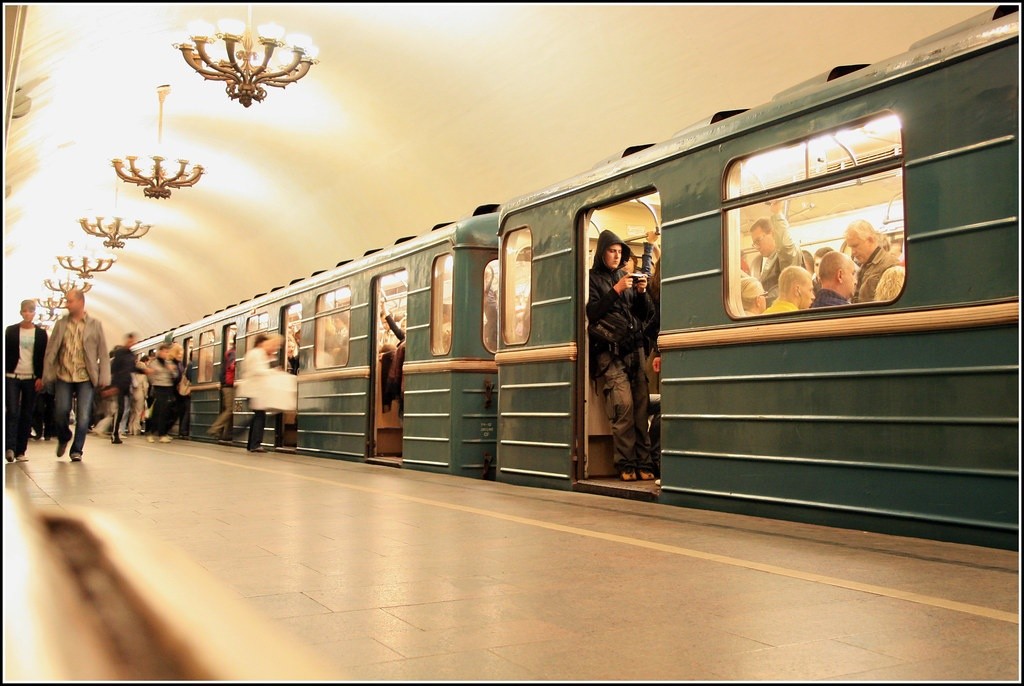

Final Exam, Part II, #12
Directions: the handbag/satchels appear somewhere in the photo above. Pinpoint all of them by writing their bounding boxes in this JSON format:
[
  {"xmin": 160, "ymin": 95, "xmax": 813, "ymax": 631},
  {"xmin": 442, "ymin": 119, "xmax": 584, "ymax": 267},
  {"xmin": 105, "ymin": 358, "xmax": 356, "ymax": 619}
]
[
  {"xmin": 587, "ymin": 311, "xmax": 629, "ymax": 345},
  {"xmin": 178, "ymin": 368, "xmax": 192, "ymax": 396}
]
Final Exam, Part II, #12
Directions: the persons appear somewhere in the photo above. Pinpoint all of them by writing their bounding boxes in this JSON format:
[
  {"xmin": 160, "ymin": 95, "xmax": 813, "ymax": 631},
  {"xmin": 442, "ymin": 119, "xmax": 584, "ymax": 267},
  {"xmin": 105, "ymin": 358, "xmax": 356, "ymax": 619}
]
[
  {"xmin": 381, "ymin": 303, "xmax": 407, "ymax": 420},
  {"xmin": 741, "ymin": 199, "xmax": 905, "ymax": 316},
  {"xmin": 42, "ymin": 289, "xmax": 111, "ymax": 461},
  {"xmin": 586, "ymin": 229, "xmax": 655, "ymax": 481},
  {"xmin": 30, "ymin": 332, "xmax": 193, "ymax": 443},
  {"xmin": 485, "ymin": 265, "xmax": 530, "ymax": 346},
  {"xmin": 624, "ymin": 231, "xmax": 662, "ymax": 485},
  {"xmin": 5, "ymin": 300, "xmax": 50, "ymax": 462},
  {"xmin": 324, "ymin": 310, "xmax": 349, "ymax": 366},
  {"xmin": 206, "ymin": 325, "xmax": 301, "ymax": 453},
  {"xmin": 443, "ymin": 303, "xmax": 451, "ymax": 352}
]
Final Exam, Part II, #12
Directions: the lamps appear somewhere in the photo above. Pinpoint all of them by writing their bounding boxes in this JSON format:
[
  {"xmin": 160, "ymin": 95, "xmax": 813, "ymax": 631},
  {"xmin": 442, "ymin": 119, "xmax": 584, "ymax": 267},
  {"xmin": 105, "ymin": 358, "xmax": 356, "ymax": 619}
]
[
  {"xmin": 170, "ymin": 6, "xmax": 320, "ymax": 107},
  {"xmin": 32, "ymin": 243, "xmax": 118, "ymax": 321},
  {"xmin": 109, "ymin": 86, "xmax": 205, "ymax": 199},
  {"xmin": 77, "ymin": 207, "xmax": 158, "ymax": 247}
]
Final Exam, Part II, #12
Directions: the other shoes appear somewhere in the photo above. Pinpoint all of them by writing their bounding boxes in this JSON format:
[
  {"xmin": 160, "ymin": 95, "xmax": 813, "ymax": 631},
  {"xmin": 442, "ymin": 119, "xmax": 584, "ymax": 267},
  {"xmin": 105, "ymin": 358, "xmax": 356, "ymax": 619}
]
[
  {"xmin": 619, "ymin": 467, "xmax": 637, "ymax": 481},
  {"xmin": 212, "ymin": 433, "xmax": 220, "ymax": 437},
  {"xmin": 5, "ymin": 449, "xmax": 14, "ymax": 462},
  {"xmin": 223, "ymin": 436, "xmax": 233, "ymax": 441},
  {"xmin": 87, "ymin": 429, "xmax": 92, "ymax": 433},
  {"xmin": 119, "ymin": 433, "xmax": 127, "ymax": 439},
  {"xmin": 70, "ymin": 452, "xmax": 82, "ymax": 460},
  {"xmin": 92, "ymin": 428, "xmax": 109, "ymax": 438},
  {"xmin": 45, "ymin": 436, "xmax": 50, "ymax": 441},
  {"xmin": 114, "ymin": 432, "xmax": 120, "ymax": 442},
  {"xmin": 638, "ymin": 468, "xmax": 655, "ymax": 480},
  {"xmin": 33, "ymin": 433, "xmax": 42, "ymax": 440},
  {"xmin": 159, "ymin": 437, "xmax": 171, "ymax": 443},
  {"xmin": 165, "ymin": 435, "xmax": 173, "ymax": 440},
  {"xmin": 57, "ymin": 443, "xmax": 67, "ymax": 457},
  {"xmin": 146, "ymin": 435, "xmax": 154, "ymax": 443},
  {"xmin": 251, "ymin": 444, "xmax": 266, "ymax": 453},
  {"xmin": 179, "ymin": 435, "xmax": 192, "ymax": 441},
  {"xmin": 17, "ymin": 455, "xmax": 29, "ymax": 461}
]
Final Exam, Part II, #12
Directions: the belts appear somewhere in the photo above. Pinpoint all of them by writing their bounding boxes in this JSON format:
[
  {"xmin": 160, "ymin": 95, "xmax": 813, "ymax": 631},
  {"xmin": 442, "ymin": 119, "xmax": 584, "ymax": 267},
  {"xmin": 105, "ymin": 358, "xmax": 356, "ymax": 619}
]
[{"xmin": 5, "ymin": 373, "xmax": 36, "ymax": 379}]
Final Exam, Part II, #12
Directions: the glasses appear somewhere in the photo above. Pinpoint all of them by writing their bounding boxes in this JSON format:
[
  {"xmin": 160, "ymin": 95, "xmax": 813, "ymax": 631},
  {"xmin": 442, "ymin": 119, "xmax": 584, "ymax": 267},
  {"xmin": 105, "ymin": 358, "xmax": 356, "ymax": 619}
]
[
  {"xmin": 751, "ymin": 229, "xmax": 770, "ymax": 248},
  {"xmin": 760, "ymin": 290, "xmax": 770, "ymax": 298}
]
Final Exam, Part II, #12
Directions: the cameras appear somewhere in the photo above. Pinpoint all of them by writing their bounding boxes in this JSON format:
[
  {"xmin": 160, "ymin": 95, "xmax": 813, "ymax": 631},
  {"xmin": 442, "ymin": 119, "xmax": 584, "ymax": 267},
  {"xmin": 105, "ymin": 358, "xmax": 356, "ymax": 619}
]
[{"xmin": 629, "ymin": 274, "xmax": 643, "ymax": 284}]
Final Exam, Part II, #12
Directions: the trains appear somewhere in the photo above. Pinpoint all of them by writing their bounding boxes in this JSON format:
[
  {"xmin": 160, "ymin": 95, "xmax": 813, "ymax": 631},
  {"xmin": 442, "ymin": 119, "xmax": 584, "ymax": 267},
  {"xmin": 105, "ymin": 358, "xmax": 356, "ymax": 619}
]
[{"xmin": 115, "ymin": 4, "xmax": 1021, "ymax": 559}]
